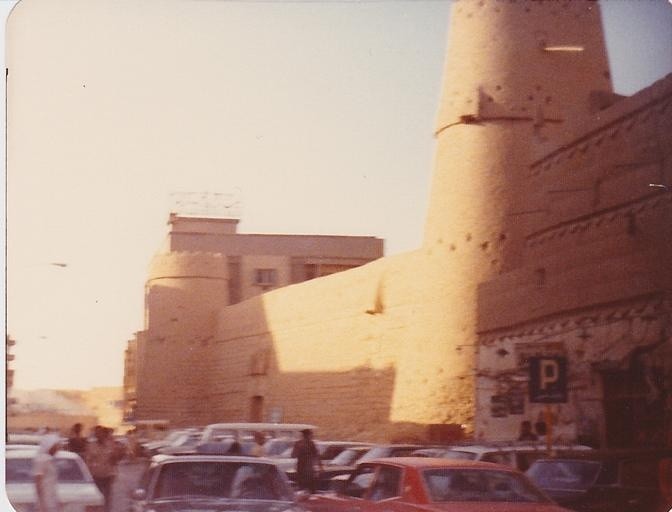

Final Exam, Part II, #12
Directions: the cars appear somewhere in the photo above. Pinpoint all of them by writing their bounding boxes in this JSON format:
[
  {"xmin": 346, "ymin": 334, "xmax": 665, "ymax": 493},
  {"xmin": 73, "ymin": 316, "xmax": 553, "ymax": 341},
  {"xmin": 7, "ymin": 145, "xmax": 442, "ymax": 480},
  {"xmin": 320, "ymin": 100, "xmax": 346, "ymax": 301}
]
[
  {"xmin": 523, "ymin": 445, "xmax": 672, "ymax": 512},
  {"xmin": 5, "ymin": 451, "xmax": 108, "ymax": 512},
  {"xmin": 4, "ymin": 433, "xmax": 73, "ymax": 453},
  {"xmin": 113, "ymin": 417, "xmax": 594, "ymax": 475},
  {"xmin": 126, "ymin": 453, "xmax": 309, "ymax": 512},
  {"xmin": 295, "ymin": 457, "xmax": 572, "ymax": 511}
]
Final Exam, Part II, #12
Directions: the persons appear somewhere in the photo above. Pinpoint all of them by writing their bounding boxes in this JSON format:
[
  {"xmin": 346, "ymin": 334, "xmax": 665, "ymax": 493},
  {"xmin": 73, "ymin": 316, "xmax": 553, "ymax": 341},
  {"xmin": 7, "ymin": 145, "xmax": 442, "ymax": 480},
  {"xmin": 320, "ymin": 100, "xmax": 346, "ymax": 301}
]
[
  {"xmin": 517, "ymin": 420, "xmax": 547, "ymax": 442},
  {"xmin": 291, "ymin": 429, "xmax": 319, "ymax": 492},
  {"xmin": 228, "ymin": 429, "xmax": 265, "ymax": 456},
  {"xmin": 30, "ymin": 433, "xmax": 62, "ymax": 512},
  {"xmin": 67, "ymin": 423, "xmax": 123, "ymax": 512}
]
